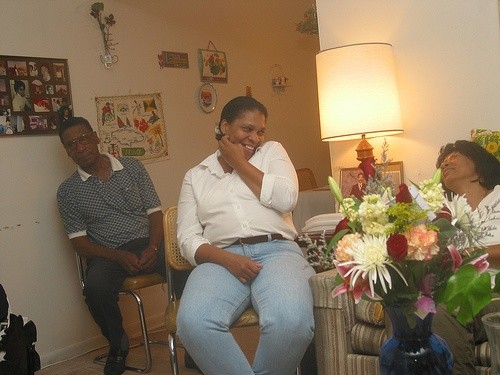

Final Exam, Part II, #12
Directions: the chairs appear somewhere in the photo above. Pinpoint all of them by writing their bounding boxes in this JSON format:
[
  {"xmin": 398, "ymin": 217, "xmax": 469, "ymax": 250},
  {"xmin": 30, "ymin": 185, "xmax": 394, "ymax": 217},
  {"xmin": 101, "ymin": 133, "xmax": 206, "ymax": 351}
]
[{"xmin": 74, "ymin": 203, "xmax": 301, "ymax": 375}]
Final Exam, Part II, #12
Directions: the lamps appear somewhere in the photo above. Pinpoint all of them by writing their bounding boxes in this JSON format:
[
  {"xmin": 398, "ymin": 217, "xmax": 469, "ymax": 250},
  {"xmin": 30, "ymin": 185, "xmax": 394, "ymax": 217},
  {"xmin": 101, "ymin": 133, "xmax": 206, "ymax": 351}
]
[{"xmin": 315, "ymin": 42, "xmax": 405, "ymax": 181}]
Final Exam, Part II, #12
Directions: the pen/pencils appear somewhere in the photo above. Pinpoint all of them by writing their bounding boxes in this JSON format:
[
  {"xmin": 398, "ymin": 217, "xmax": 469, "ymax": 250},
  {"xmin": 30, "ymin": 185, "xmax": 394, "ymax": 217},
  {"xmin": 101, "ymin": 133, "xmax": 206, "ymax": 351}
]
[{"xmin": 121, "ymin": 187, "xmax": 123, "ymax": 191}]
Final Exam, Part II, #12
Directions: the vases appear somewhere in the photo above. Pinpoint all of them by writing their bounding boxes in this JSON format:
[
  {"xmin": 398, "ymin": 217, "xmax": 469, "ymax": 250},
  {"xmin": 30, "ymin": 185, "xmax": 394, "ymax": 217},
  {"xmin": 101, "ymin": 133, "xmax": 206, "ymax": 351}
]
[{"xmin": 376, "ymin": 300, "xmax": 454, "ymax": 374}]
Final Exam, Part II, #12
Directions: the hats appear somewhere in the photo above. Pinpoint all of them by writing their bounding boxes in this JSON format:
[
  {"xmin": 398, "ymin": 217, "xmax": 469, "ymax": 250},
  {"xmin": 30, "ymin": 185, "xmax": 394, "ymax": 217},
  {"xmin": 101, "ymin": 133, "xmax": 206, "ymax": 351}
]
[{"xmin": 31, "ymin": 80, "xmax": 42, "ymax": 86}]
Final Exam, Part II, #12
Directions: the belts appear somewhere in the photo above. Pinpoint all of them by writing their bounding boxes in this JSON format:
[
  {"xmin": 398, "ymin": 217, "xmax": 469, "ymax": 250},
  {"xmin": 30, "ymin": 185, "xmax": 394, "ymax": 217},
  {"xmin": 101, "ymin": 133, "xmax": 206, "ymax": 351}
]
[{"xmin": 231, "ymin": 233, "xmax": 288, "ymax": 245}]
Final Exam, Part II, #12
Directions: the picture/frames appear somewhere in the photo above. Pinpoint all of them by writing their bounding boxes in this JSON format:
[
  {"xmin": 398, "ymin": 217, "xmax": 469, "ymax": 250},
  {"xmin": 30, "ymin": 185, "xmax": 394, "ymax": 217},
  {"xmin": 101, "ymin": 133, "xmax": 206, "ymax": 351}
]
[
  {"xmin": 0, "ymin": 54, "xmax": 73, "ymax": 136},
  {"xmin": 337, "ymin": 161, "xmax": 404, "ymax": 211}
]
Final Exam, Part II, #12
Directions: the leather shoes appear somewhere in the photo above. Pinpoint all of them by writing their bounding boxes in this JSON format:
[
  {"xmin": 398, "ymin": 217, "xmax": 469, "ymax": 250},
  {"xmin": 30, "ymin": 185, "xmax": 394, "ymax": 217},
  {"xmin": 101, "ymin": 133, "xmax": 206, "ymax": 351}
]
[{"xmin": 104, "ymin": 338, "xmax": 130, "ymax": 375}]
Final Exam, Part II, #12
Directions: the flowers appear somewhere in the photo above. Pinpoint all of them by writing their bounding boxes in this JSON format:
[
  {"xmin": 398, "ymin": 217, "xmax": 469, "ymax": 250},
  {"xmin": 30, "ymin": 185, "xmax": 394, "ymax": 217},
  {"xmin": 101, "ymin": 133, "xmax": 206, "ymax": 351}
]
[{"xmin": 326, "ymin": 135, "xmax": 500, "ymax": 324}]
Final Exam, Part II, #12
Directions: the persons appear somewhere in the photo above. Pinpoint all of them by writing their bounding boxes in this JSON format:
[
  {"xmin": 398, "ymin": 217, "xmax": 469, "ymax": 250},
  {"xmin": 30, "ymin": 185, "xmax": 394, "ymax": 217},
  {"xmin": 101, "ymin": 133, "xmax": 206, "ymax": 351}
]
[
  {"xmin": 56, "ymin": 117, "xmax": 198, "ymax": 375},
  {"xmin": 177, "ymin": 95, "xmax": 317, "ymax": 375},
  {"xmin": 0, "ymin": 63, "xmax": 72, "ymax": 135},
  {"xmin": 430, "ymin": 140, "xmax": 500, "ymax": 375}
]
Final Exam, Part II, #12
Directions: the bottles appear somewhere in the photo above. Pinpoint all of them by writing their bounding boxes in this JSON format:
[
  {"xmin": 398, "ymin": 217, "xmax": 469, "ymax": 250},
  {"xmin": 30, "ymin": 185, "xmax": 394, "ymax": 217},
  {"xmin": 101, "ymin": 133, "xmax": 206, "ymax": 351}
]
[{"xmin": 480, "ymin": 311, "xmax": 500, "ymax": 375}]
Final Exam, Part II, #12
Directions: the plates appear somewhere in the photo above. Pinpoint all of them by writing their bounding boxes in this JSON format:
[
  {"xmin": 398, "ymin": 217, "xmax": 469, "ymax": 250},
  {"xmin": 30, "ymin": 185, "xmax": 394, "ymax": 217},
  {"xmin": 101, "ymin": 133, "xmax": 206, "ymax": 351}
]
[{"xmin": 199, "ymin": 83, "xmax": 217, "ymax": 113}]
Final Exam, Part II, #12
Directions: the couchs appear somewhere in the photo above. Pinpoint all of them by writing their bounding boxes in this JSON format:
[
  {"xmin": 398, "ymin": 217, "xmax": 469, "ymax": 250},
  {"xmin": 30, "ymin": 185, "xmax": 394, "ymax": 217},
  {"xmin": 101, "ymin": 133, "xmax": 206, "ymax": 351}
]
[{"xmin": 307, "ymin": 265, "xmax": 495, "ymax": 375}]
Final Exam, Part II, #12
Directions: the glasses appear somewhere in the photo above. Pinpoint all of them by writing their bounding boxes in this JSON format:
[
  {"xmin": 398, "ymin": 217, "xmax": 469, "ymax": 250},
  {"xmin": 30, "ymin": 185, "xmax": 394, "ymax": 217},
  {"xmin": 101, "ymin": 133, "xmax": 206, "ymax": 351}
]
[
  {"xmin": 64, "ymin": 130, "xmax": 93, "ymax": 150},
  {"xmin": 20, "ymin": 89, "xmax": 25, "ymax": 91}
]
